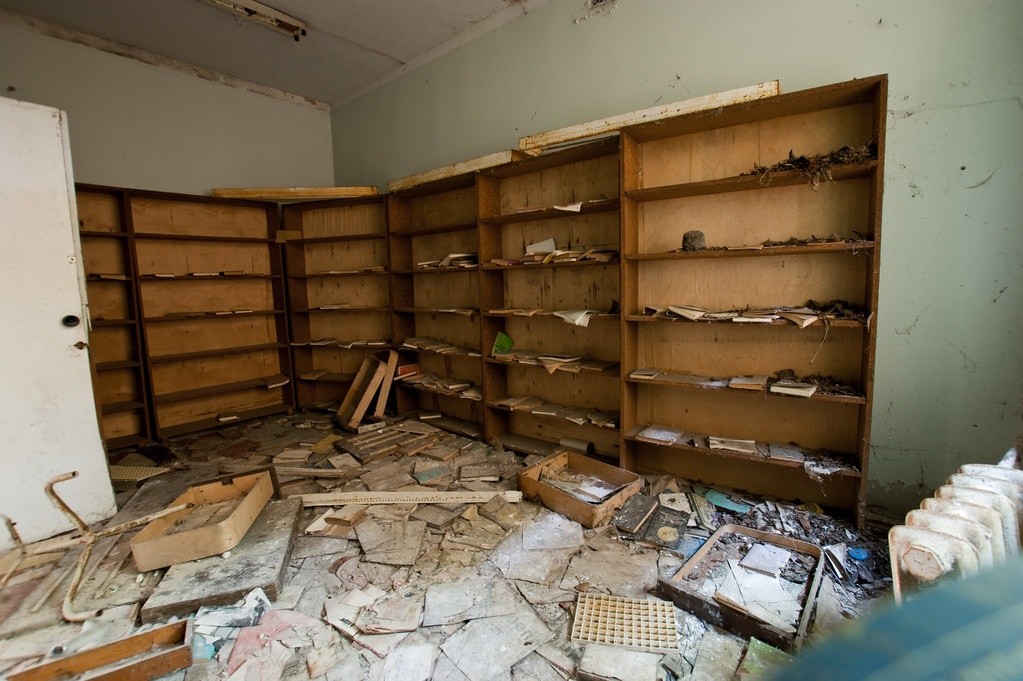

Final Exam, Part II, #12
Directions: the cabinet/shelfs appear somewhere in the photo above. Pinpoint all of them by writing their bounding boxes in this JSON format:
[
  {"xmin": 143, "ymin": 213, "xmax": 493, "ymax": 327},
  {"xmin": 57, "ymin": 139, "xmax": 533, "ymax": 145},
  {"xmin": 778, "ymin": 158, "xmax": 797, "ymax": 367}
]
[{"xmin": 73, "ymin": 70, "xmax": 889, "ymax": 535}]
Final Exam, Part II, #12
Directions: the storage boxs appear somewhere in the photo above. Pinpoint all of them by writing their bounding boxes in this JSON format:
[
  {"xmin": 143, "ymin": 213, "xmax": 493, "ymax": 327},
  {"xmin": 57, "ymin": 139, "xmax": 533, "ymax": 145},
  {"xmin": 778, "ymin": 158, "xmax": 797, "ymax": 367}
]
[
  {"xmin": 333, "ymin": 356, "xmax": 387, "ymax": 433},
  {"xmin": 516, "ymin": 448, "xmax": 641, "ymax": 529},
  {"xmin": 128, "ymin": 466, "xmax": 281, "ymax": 574},
  {"xmin": 364, "ymin": 349, "xmax": 398, "ymax": 423},
  {"xmin": 656, "ymin": 523, "xmax": 825, "ymax": 656}
]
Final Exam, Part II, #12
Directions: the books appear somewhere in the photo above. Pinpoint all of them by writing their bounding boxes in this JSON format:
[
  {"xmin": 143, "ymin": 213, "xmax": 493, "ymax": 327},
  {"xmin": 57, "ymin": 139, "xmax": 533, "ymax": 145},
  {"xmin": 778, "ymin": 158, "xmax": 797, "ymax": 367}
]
[
  {"xmin": 262, "ymin": 373, "xmax": 291, "ymax": 390},
  {"xmin": 516, "ymin": 192, "xmax": 608, "ymax": 213},
  {"xmin": 218, "ymin": 410, "xmax": 239, "ymax": 422},
  {"xmin": 289, "ymin": 266, "xmax": 389, "ymax": 349},
  {"xmin": 89, "ymin": 270, "xmax": 265, "ymax": 280},
  {"xmin": 299, "ymin": 369, "xmax": 329, "ymax": 381},
  {"xmin": 489, "ymin": 395, "xmax": 621, "ymax": 427},
  {"xmin": 644, "ymin": 302, "xmax": 837, "ymax": 327},
  {"xmin": 166, "ymin": 310, "xmax": 253, "ymax": 316},
  {"xmin": 400, "ymin": 336, "xmax": 482, "ymax": 359},
  {"xmin": 634, "ymin": 424, "xmax": 807, "ymax": 464},
  {"xmin": 393, "ymin": 363, "xmax": 482, "ymax": 401},
  {"xmin": 489, "ymin": 330, "xmax": 620, "ymax": 374},
  {"xmin": 629, "ymin": 368, "xmax": 660, "ymax": 380},
  {"xmin": 436, "ymin": 307, "xmax": 603, "ymax": 326},
  {"xmin": 729, "ymin": 375, "xmax": 819, "ymax": 398},
  {"xmin": 415, "ymin": 238, "xmax": 617, "ymax": 269}
]
